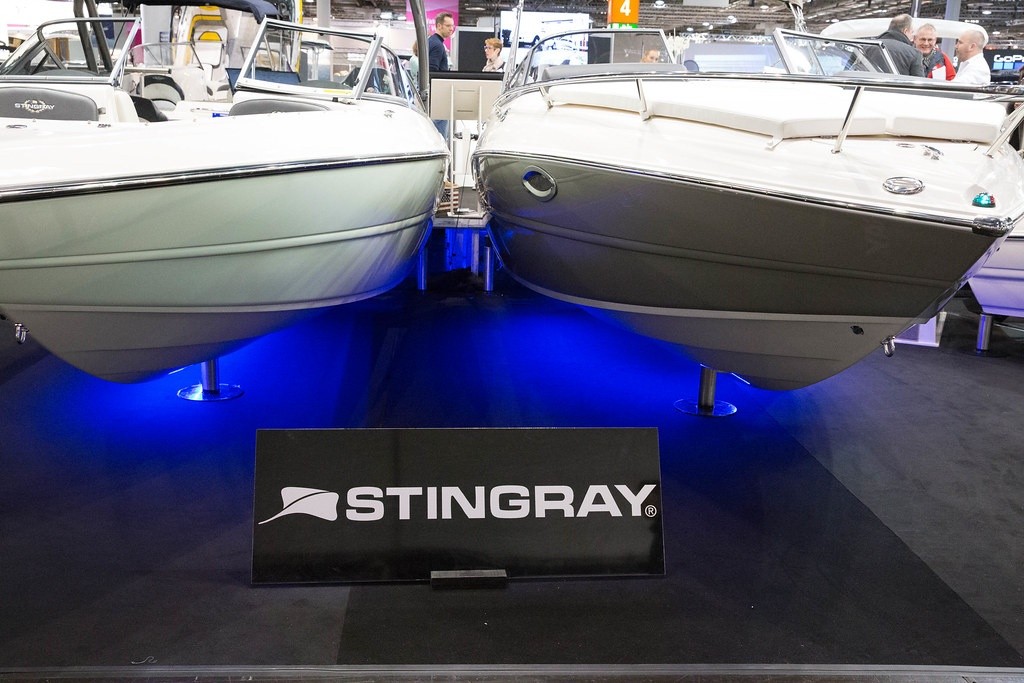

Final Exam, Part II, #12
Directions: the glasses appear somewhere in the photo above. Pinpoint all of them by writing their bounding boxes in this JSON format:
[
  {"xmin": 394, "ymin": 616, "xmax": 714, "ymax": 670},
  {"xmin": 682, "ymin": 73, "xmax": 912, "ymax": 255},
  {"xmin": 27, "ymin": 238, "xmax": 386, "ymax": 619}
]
[
  {"xmin": 444, "ymin": 23, "xmax": 454, "ymax": 28},
  {"xmin": 484, "ymin": 46, "xmax": 497, "ymax": 50}
]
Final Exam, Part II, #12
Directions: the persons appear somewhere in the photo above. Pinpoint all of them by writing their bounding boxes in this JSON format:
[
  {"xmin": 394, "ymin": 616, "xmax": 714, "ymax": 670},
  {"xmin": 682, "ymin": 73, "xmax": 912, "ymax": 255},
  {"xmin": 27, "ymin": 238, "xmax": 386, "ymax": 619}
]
[
  {"xmin": 640, "ymin": 49, "xmax": 660, "ymax": 63},
  {"xmin": 913, "ymin": 24, "xmax": 956, "ymax": 82},
  {"xmin": 428, "ymin": 12, "xmax": 458, "ymax": 189},
  {"xmin": 950, "ymin": 30, "xmax": 991, "ymax": 85},
  {"xmin": 849, "ymin": 14, "xmax": 925, "ymax": 78},
  {"xmin": 409, "ymin": 39, "xmax": 419, "ymax": 102},
  {"xmin": 471, "ymin": 38, "xmax": 509, "ymax": 191}
]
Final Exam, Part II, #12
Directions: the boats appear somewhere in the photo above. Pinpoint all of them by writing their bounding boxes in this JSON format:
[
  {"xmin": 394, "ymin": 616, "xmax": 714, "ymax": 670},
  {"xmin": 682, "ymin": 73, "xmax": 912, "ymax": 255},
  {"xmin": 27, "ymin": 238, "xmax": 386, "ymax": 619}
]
[
  {"xmin": 473, "ymin": 0, "xmax": 1024, "ymax": 393},
  {"xmin": 0, "ymin": 3, "xmax": 450, "ymax": 385}
]
